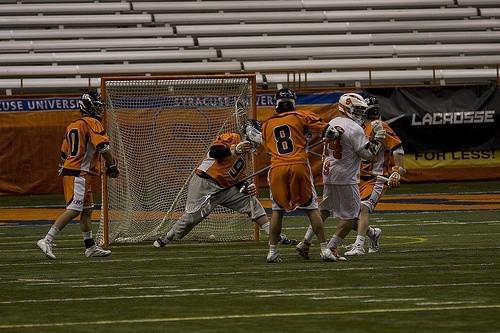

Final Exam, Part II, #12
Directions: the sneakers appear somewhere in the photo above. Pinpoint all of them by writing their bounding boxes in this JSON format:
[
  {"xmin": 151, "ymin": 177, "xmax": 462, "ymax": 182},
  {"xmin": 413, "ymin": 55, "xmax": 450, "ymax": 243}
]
[
  {"xmin": 295, "ymin": 240, "xmax": 311, "ymax": 259},
  {"xmin": 36, "ymin": 239, "xmax": 57, "ymax": 259},
  {"xmin": 278, "ymin": 236, "xmax": 292, "ymax": 246},
  {"xmin": 153, "ymin": 232, "xmax": 173, "ymax": 248},
  {"xmin": 267, "ymin": 253, "xmax": 282, "ymax": 263},
  {"xmin": 330, "ymin": 248, "xmax": 347, "ymax": 261},
  {"xmin": 344, "ymin": 243, "xmax": 365, "ymax": 255},
  {"xmin": 369, "ymin": 228, "xmax": 382, "ymax": 253},
  {"xmin": 321, "ymin": 248, "xmax": 336, "ymax": 261},
  {"xmin": 85, "ymin": 245, "xmax": 112, "ymax": 257}
]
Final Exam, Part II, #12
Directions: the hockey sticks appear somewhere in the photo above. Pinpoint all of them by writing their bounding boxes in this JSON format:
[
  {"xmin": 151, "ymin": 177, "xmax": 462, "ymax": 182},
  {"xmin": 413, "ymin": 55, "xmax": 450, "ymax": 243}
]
[
  {"xmin": 245, "ymin": 125, "xmax": 402, "ymax": 187},
  {"xmin": 56, "ymin": 161, "xmax": 117, "ymax": 178},
  {"xmin": 185, "ymin": 139, "xmax": 326, "ymax": 214},
  {"xmin": 235, "ymin": 98, "xmax": 256, "ymax": 221}
]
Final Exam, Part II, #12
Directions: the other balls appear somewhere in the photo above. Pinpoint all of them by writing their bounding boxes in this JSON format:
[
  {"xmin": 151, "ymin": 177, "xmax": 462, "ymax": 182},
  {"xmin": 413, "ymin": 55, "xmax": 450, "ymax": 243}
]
[{"xmin": 209, "ymin": 235, "xmax": 215, "ymax": 239}]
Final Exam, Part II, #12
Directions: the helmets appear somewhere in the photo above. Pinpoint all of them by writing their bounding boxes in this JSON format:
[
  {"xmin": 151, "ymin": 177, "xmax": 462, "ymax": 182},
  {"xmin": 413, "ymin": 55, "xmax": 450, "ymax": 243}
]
[
  {"xmin": 79, "ymin": 91, "xmax": 106, "ymax": 122},
  {"xmin": 239, "ymin": 118, "xmax": 263, "ymax": 149},
  {"xmin": 275, "ymin": 87, "xmax": 297, "ymax": 114},
  {"xmin": 338, "ymin": 93, "xmax": 368, "ymax": 126},
  {"xmin": 362, "ymin": 95, "xmax": 380, "ymax": 119}
]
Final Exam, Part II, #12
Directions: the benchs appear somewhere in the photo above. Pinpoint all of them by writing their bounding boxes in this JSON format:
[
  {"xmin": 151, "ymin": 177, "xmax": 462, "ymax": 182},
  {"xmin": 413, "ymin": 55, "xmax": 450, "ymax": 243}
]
[{"xmin": 0, "ymin": 0, "xmax": 500, "ymax": 89}]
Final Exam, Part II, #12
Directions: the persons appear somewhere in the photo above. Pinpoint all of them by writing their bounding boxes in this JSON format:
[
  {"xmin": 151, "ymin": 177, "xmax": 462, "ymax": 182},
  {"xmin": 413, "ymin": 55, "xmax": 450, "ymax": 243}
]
[
  {"xmin": 153, "ymin": 87, "xmax": 406, "ymax": 262},
  {"xmin": 36, "ymin": 93, "xmax": 119, "ymax": 261}
]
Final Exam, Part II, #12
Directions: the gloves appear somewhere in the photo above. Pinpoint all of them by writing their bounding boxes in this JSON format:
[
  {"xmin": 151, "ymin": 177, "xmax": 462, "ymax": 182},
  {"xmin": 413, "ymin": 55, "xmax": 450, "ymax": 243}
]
[
  {"xmin": 230, "ymin": 141, "xmax": 250, "ymax": 157},
  {"xmin": 105, "ymin": 160, "xmax": 120, "ymax": 178},
  {"xmin": 387, "ymin": 166, "xmax": 407, "ymax": 189},
  {"xmin": 247, "ymin": 183, "xmax": 257, "ymax": 196},
  {"xmin": 371, "ymin": 120, "xmax": 386, "ymax": 139},
  {"xmin": 335, "ymin": 126, "xmax": 345, "ymax": 136}
]
